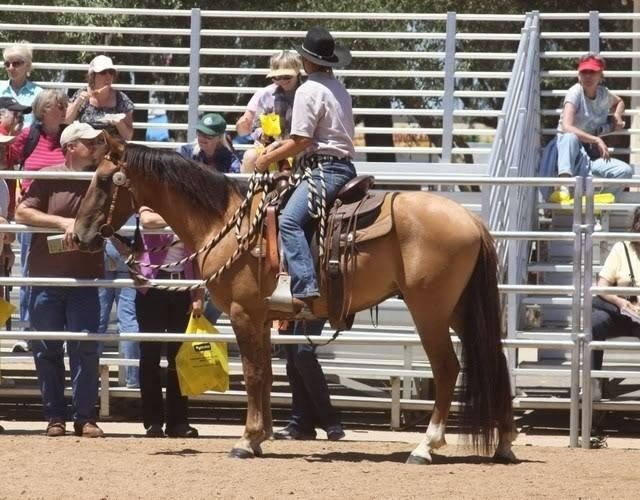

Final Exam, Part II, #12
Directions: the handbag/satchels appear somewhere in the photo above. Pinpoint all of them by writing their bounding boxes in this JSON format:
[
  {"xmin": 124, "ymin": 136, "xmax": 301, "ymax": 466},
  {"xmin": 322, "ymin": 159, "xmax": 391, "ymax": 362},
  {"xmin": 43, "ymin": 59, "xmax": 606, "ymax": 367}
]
[{"xmin": 592, "ymin": 294, "xmax": 637, "ymax": 323}]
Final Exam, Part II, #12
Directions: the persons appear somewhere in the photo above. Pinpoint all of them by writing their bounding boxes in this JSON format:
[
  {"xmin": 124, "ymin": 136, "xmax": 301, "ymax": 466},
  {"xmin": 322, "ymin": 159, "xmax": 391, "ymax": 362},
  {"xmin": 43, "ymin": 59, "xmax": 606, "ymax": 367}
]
[
  {"xmin": 134, "ymin": 205, "xmax": 205, "ymax": 438},
  {"xmin": 578, "ymin": 206, "xmax": 640, "ymax": 402},
  {"xmin": 538, "ymin": 52, "xmax": 632, "ymax": 214},
  {"xmin": 0, "ymin": 38, "xmax": 243, "ymax": 390},
  {"xmin": 253, "ymin": 26, "xmax": 357, "ymax": 301},
  {"xmin": 14, "ymin": 122, "xmax": 107, "ymax": 437},
  {"xmin": 236, "ymin": 50, "xmax": 302, "ymax": 174},
  {"xmin": 273, "ymin": 318, "xmax": 345, "ymax": 441}
]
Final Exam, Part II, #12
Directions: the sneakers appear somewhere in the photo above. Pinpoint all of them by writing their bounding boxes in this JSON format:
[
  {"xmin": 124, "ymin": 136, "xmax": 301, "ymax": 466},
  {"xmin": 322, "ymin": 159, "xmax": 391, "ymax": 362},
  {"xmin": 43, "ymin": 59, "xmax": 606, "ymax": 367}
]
[
  {"xmin": 549, "ymin": 185, "xmax": 570, "ymax": 203},
  {"xmin": 263, "ymin": 291, "xmax": 320, "ymax": 299},
  {"xmin": 13, "ymin": 343, "xmax": 28, "ymax": 353},
  {"xmin": 579, "ymin": 378, "xmax": 604, "ymax": 402},
  {"xmin": 326, "ymin": 423, "xmax": 346, "ymax": 440},
  {"xmin": 271, "ymin": 420, "xmax": 317, "ymax": 440},
  {"xmin": 147, "ymin": 424, "xmax": 165, "ymax": 437},
  {"xmin": 166, "ymin": 422, "xmax": 198, "ymax": 437},
  {"xmin": 73, "ymin": 421, "xmax": 104, "ymax": 437},
  {"xmin": 47, "ymin": 422, "xmax": 65, "ymax": 436}
]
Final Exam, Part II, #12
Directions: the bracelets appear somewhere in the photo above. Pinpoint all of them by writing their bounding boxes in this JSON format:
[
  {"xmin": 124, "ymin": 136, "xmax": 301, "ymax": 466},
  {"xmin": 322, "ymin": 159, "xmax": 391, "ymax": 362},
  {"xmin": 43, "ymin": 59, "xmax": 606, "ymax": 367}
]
[{"xmin": 271, "ymin": 140, "xmax": 280, "ymax": 146}]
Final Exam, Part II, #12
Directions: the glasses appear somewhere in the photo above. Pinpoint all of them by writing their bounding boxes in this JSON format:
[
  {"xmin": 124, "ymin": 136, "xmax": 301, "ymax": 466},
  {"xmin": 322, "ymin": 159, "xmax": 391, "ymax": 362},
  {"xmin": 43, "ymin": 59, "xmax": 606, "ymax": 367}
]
[
  {"xmin": 99, "ymin": 70, "xmax": 114, "ymax": 75},
  {"xmin": 4, "ymin": 61, "xmax": 25, "ymax": 68},
  {"xmin": 271, "ymin": 75, "xmax": 293, "ymax": 81}
]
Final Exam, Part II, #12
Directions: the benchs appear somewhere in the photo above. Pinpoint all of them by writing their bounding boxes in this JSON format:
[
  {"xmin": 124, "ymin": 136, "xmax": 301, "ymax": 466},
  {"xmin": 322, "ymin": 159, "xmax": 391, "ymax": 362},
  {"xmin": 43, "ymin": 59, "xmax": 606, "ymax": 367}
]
[{"xmin": 517, "ymin": 192, "xmax": 640, "ymax": 413}]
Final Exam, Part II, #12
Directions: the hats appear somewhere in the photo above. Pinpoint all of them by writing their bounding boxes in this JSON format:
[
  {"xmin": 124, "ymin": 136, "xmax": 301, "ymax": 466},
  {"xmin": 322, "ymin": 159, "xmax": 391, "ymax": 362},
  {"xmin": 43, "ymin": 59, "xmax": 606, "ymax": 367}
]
[
  {"xmin": 60, "ymin": 120, "xmax": 103, "ymax": 148},
  {"xmin": 194, "ymin": 113, "xmax": 227, "ymax": 137},
  {"xmin": 0, "ymin": 96, "xmax": 32, "ymax": 114},
  {"xmin": 265, "ymin": 60, "xmax": 302, "ymax": 79},
  {"xmin": 88, "ymin": 54, "xmax": 116, "ymax": 76},
  {"xmin": 296, "ymin": 27, "xmax": 353, "ymax": 67},
  {"xmin": 579, "ymin": 54, "xmax": 605, "ymax": 73}
]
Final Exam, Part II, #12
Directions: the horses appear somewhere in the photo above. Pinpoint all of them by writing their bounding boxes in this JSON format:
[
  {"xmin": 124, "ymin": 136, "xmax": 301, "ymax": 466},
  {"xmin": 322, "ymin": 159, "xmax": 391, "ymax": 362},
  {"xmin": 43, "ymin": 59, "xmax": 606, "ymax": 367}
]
[{"xmin": 73, "ymin": 123, "xmax": 519, "ymax": 464}]
